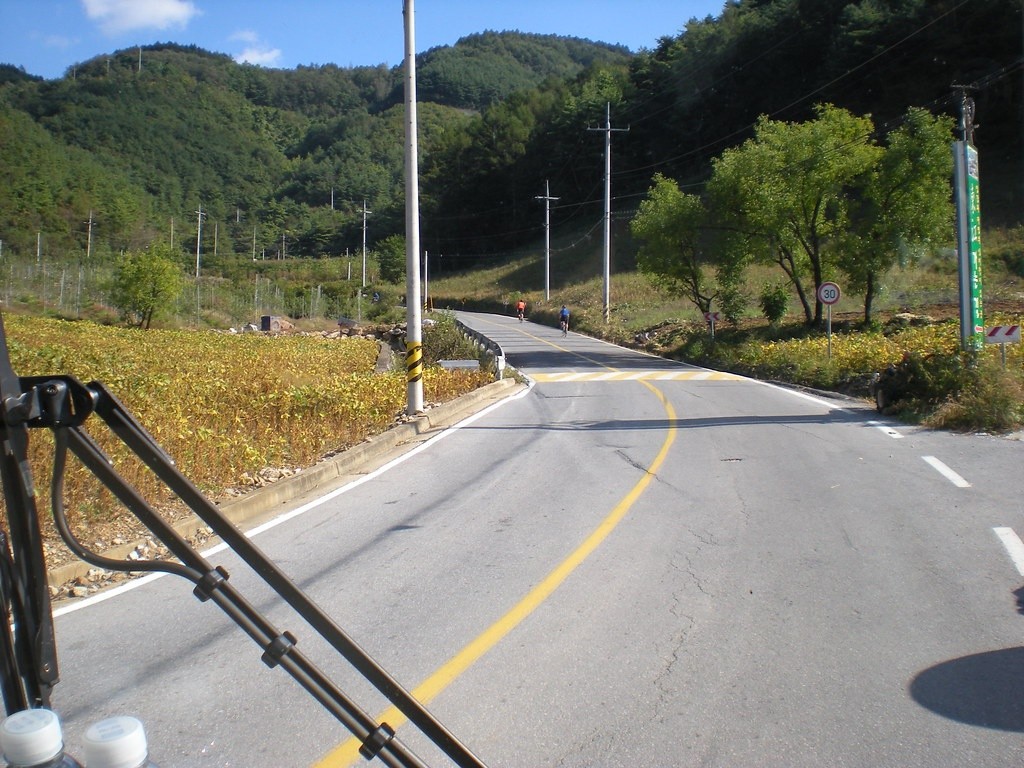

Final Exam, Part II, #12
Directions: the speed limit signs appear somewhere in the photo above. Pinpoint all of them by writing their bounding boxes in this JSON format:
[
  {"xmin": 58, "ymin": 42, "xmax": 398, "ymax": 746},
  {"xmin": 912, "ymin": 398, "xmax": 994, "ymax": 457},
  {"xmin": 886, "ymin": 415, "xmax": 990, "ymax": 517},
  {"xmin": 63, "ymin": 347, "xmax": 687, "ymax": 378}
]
[{"xmin": 817, "ymin": 281, "xmax": 841, "ymax": 305}]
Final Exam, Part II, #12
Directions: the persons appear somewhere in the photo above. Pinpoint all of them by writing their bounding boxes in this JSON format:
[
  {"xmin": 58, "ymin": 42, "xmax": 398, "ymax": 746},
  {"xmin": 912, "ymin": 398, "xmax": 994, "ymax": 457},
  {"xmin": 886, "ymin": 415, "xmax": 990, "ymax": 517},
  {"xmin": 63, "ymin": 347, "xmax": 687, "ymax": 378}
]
[
  {"xmin": 516, "ymin": 300, "xmax": 526, "ymax": 320},
  {"xmin": 560, "ymin": 305, "xmax": 570, "ymax": 335},
  {"xmin": 374, "ymin": 293, "xmax": 379, "ymax": 301}
]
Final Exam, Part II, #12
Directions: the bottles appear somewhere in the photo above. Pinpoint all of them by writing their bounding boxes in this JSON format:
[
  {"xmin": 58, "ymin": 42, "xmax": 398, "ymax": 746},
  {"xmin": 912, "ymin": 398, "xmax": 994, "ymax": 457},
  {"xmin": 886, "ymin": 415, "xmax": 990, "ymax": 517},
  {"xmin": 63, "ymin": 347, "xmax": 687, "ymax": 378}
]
[
  {"xmin": 83, "ymin": 715, "xmax": 161, "ymax": 768},
  {"xmin": 0, "ymin": 709, "xmax": 84, "ymax": 768}
]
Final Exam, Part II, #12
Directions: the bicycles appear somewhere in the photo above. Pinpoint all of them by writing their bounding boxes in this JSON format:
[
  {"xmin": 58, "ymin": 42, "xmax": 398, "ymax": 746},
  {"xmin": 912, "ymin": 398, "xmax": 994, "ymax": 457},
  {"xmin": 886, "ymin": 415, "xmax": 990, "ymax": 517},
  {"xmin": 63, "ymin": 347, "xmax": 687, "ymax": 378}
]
[
  {"xmin": 517, "ymin": 308, "xmax": 525, "ymax": 322},
  {"xmin": 561, "ymin": 321, "xmax": 569, "ymax": 336}
]
